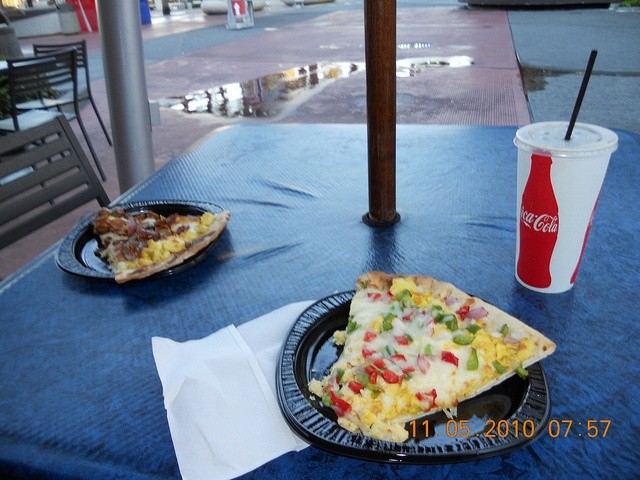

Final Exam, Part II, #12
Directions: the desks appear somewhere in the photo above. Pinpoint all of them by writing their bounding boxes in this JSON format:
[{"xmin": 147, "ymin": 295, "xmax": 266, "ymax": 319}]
[
  {"xmin": 0, "ymin": 55, "xmax": 58, "ymax": 78},
  {"xmin": 1, "ymin": 121, "xmax": 640, "ymax": 478}
]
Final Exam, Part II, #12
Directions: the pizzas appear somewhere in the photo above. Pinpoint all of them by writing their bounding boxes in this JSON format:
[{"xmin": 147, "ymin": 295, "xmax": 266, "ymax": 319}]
[
  {"xmin": 312, "ymin": 269, "xmax": 559, "ymax": 445},
  {"xmin": 94, "ymin": 205, "xmax": 230, "ymax": 283}
]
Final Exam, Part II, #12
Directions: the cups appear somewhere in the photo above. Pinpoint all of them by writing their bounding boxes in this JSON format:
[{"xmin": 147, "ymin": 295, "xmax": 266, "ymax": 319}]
[{"xmin": 512, "ymin": 120, "xmax": 619, "ymax": 295}]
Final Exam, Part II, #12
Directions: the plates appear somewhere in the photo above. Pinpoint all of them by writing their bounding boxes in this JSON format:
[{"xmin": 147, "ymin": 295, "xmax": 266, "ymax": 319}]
[
  {"xmin": 53, "ymin": 198, "xmax": 227, "ymax": 283},
  {"xmin": 274, "ymin": 290, "xmax": 552, "ymax": 465}
]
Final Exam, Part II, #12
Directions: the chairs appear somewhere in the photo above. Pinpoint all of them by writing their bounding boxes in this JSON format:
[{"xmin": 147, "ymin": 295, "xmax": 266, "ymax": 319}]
[
  {"xmin": 13, "ymin": 37, "xmax": 114, "ymax": 148},
  {"xmin": 5, "ymin": 47, "xmax": 107, "ymax": 184},
  {"xmin": 0, "ymin": 113, "xmax": 113, "ymax": 281}
]
